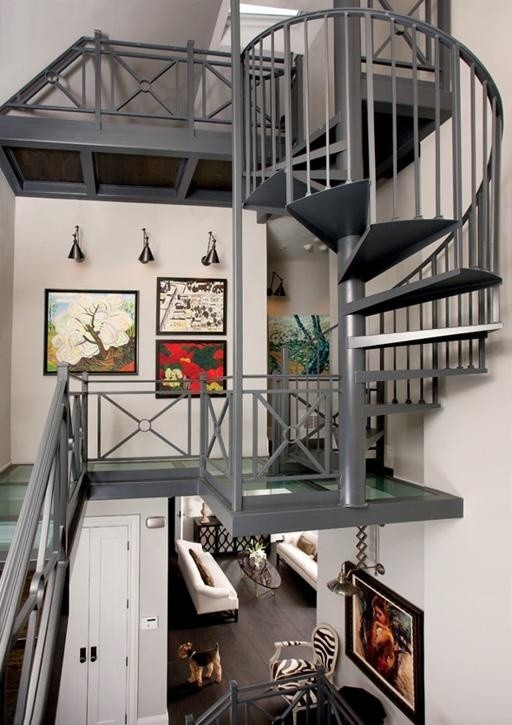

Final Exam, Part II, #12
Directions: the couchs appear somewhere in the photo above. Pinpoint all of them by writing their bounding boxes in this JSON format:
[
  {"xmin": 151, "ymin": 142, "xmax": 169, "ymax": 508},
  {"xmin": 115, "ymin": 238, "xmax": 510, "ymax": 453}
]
[
  {"xmin": 276, "ymin": 531, "xmax": 318, "ymax": 592},
  {"xmin": 175, "ymin": 539, "xmax": 240, "ymax": 623}
]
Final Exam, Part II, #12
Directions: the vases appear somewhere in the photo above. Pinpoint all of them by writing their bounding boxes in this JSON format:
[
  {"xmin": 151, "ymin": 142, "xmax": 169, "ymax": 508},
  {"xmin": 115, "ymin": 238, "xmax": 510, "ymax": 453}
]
[{"xmin": 249, "ymin": 557, "xmax": 265, "ymax": 570}]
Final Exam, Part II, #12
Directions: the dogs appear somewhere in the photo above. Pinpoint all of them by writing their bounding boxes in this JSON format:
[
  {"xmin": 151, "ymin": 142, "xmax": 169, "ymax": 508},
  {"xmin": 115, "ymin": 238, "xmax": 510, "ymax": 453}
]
[{"xmin": 178, "ymin": 640, "xmax": 222, "ymax": 687}]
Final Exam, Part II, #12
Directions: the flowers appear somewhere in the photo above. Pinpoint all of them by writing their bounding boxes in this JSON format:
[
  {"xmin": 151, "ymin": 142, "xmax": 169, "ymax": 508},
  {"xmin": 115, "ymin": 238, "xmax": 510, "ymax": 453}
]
[{"xmin": 248, "ymin": 542, "xmax": 267, "ymax": 559}]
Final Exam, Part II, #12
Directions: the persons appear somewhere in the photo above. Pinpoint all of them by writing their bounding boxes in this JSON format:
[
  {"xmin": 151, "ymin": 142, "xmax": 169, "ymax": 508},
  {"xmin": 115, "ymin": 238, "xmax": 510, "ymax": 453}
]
[
  {"xmin": 390, "ymin": 612, "xmax": 404, "ymax": 642},
  {"xmin": 368, "ymin": 595, "xmax": 397, "ymax": 681}
]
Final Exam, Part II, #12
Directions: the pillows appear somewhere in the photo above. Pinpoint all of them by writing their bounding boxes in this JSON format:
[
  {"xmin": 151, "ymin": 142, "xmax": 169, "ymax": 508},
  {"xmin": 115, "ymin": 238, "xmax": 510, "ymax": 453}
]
[
  {"xmin": 188, "ymin": 549, "xmax": 214, "ymax": 587},
  {"xmin": 297, "ymin": 536, "xmax": 316, "ymax": 555}
]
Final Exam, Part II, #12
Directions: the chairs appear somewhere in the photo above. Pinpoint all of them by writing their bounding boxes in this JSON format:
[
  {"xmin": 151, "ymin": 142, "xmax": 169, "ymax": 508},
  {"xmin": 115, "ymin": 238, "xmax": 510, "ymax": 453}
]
[{"xmin": 267, "ymin": 622, "xmax": 340, "ymax": 725}]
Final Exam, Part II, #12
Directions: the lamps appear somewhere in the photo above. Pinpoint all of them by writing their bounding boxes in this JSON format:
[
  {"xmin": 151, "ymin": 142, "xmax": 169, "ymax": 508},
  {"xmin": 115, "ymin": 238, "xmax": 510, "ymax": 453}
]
[
  {"xmin": 267, "ymin": 271, "xmax": 286, "ymax": 297},
  {"xmin": 327, "ymin": 561, "xmax": 386, "ymax": 597},
  {"xmin": 67, "ymin": 225, "xmax": 220, "ymax": 266}
]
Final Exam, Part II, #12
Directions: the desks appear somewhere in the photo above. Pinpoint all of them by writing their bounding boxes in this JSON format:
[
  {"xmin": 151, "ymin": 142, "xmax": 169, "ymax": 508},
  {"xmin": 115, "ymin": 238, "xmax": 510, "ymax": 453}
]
[{"xmin": 194, "ymin": 516, "xmax": 271, "ymax": 554}]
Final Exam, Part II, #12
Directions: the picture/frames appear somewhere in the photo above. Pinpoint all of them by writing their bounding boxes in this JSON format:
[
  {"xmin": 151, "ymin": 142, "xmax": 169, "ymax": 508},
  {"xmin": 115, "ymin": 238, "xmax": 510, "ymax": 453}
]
[
  {"xmin": 344, "ymin": 561, "xmax": 425, "ymax": 724},
  {"xmin": 43, "ymin": 276, "xmax": 228, "ymax": 400}
]
